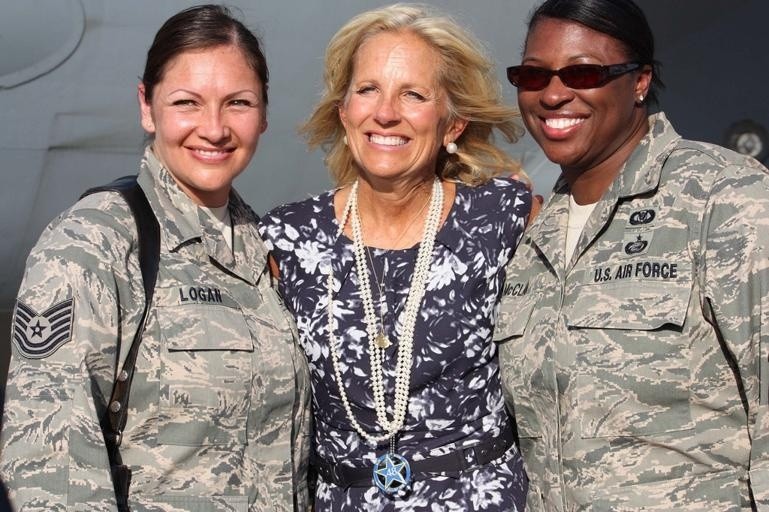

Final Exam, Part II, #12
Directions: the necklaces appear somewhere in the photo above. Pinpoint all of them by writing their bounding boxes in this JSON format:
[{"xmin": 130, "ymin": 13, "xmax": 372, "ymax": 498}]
[{"xmin": 327, "ymin": 173, "xmax": 444, "ymax": 492}]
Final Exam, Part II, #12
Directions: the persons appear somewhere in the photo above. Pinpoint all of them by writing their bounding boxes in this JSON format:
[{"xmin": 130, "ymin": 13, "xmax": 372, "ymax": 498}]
[
  {"xmin": 0, "ymin": 2, "xmax": 313, "ymax": 510},
  {"xmin": 258, "ymin": 3, "xmax": 542, "ymax": 509},
  {"xmin": 494, "ymin": 2, "xmax": 768, "ymax": 512}
]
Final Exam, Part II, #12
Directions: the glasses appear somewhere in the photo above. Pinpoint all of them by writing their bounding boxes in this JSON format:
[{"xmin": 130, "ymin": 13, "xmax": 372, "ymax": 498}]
[{"xmin": 506, "ymin": 63, "xmax": 647, "ymax": 89}]
